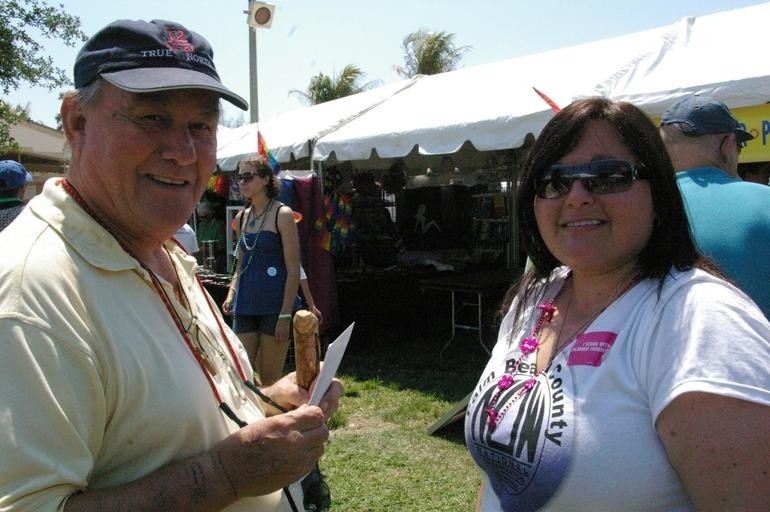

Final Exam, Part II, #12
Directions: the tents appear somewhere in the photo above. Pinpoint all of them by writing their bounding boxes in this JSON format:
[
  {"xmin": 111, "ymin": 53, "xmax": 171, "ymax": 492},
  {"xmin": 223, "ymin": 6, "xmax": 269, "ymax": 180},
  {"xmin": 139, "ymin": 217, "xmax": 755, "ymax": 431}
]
[
  {"xmin": 578, "ymin": 2, "xmax": 769, "ymax": 122},
  {"xmin": 313, "ymin": 15, "xmax": 686, "ymax": 161},
  {"xmin": 215, "ymin": 74, "xmax": 422, "ymax": 172}
]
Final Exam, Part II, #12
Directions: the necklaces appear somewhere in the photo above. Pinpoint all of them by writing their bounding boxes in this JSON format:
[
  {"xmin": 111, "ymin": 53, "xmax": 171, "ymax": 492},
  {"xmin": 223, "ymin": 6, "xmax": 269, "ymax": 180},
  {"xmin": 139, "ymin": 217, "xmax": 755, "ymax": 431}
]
[
  {"xmin": 228, "ymin": 199, "xmax": 275, "ymax": 282},
  {"xmin": 486, "ymin": 269, "xmax": 646, "ymax": 433}
]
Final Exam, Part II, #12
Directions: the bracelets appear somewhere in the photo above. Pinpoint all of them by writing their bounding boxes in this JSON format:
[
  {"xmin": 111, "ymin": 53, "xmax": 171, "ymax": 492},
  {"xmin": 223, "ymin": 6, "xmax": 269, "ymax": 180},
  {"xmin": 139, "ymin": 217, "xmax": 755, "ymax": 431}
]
[
  {"xmin": 277, "ymin": 312, "xmax": 291, "ymax": 320},
  {"xmin": 227, "ymin": 287, "xmax": 235, "ymax": 292}
]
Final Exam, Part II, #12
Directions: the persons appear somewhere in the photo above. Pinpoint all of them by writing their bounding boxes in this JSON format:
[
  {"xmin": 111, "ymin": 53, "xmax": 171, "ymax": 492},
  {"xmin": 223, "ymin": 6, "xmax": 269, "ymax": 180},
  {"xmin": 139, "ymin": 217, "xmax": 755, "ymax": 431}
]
[
  {"xmin": 175, "ymin": 223, "xmax": 198, "ymax": 259},
  {"xmin": 656, "ymin": 96, "xmax": 769, "ymax": 316},
  {"xmin": 464, "ymin": 98, "xmax": 770, "ymax": 512},
  {"xmin": 193, "ymin": 201, "xmax": 223, "ymax": 268},
  {"xmin": 294, "ymin": 263, "xmax": 322, "ymax": 325},
  {"xmin": 0, "ymin": 18, "xmax": 343, "ymax": 511},
  {"xmin": 0, "ymin": 159, "xmax": 33, "ymax": 229},
  {"xmin": 221, "ymin": 158, "xmax": 300, "ymax": 386}
]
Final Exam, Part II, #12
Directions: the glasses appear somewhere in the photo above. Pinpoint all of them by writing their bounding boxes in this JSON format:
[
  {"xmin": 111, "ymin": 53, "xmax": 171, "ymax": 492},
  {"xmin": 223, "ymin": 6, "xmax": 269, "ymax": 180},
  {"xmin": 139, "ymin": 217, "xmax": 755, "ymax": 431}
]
[
  {"xmin": 236, "ymin": 171, "xmax": 258, "ymax": 182},
  {"xmin": 531, "ymin": 158, "xmax": 650, "ymax": 199}
]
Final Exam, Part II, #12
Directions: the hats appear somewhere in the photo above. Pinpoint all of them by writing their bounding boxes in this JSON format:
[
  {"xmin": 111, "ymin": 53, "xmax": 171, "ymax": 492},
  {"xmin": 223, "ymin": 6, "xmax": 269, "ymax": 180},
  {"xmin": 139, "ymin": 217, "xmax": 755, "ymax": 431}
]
[
  {"xmin": 71, "ymin": 18, "xmax": 250, "ymax": 112},
  {"xmin": 659, "ymin": 93, "xmax": 755, "ymax": 142}
]
